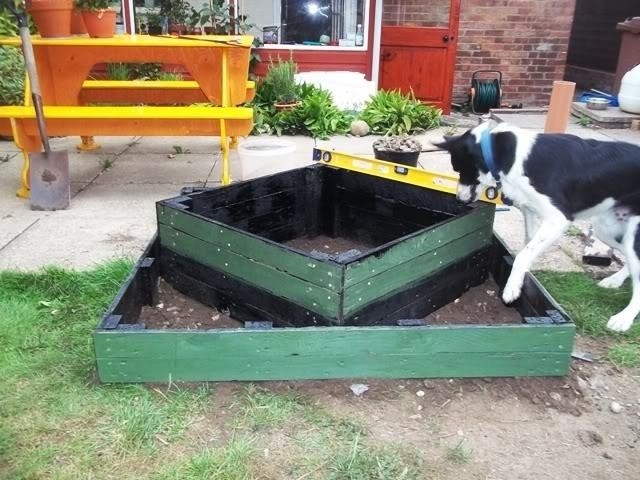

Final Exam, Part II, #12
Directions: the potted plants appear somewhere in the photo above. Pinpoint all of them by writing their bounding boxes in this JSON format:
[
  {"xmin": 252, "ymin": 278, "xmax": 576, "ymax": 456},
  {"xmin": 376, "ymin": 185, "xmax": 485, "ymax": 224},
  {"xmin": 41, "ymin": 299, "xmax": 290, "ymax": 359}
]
[
  {"xmin": 267, "ymin": 51, "xmax": 302, "ymax": 114},
  {"xmin": 367, "ymin": 87, "xmax": 423, "ymax": 168},
  {"xmin": 75, "ymin": 0, "xmax": 117, "ymax": 39}
]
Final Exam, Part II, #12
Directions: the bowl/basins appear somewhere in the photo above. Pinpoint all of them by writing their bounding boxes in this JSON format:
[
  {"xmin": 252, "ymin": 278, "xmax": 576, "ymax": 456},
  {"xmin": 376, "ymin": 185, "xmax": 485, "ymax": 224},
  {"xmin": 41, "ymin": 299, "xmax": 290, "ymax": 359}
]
[{"xmin": 585, "ymin": 97, "xmax": 611, "ymax": 110}]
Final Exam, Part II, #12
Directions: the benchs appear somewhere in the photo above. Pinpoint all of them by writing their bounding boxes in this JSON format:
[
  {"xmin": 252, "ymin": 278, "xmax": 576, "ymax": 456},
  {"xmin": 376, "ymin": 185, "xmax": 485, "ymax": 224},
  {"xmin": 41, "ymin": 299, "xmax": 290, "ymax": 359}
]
[
  {"xmin": 75, "ymin": 80, "xmax": 255, "ymax": 151},
  {"xmin": 1, "ymin": 107, "xmax": 253, "ymax": 199}
]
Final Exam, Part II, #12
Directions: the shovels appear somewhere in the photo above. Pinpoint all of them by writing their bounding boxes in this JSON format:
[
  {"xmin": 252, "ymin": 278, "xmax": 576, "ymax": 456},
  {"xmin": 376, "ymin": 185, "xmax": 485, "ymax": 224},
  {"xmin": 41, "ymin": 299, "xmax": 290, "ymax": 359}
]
[{"xmin": 1, "ymin": 0, "xmax": 70, "ymax": 211}]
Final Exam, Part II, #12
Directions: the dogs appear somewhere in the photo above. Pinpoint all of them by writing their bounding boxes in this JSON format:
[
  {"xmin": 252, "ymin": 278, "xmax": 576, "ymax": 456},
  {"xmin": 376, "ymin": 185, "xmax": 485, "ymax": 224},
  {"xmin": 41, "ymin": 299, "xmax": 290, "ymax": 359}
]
[{"xmin": 430, "ymin": 119, "xmax": 640, "ymax": 334}]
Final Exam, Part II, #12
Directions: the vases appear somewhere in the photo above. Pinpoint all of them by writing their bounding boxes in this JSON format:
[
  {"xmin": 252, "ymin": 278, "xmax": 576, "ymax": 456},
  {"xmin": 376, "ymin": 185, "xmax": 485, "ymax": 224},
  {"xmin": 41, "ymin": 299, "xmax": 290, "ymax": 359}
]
[
  {"xmin": 71, "ymin": 10, "xmax": 88, "ymax": 33},
  {"xmin": 25, "ymin": 1, "xmax": 72, "ymax": 36}
]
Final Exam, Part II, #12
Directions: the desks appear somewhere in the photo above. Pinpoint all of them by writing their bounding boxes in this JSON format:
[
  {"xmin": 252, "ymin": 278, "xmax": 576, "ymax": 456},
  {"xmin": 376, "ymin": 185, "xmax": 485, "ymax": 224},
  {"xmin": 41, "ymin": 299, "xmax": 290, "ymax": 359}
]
[{"xmin": 3, "ymin": 34, "xmax": 255, "ymax": 150}]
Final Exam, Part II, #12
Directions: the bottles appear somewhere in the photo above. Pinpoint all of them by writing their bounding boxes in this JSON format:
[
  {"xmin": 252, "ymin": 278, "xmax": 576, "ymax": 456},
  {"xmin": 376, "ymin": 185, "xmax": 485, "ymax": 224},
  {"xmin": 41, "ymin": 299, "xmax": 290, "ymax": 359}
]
[{"xmin": 355, "ymin": 24, "xmax": 363, "ymax": 46}]
